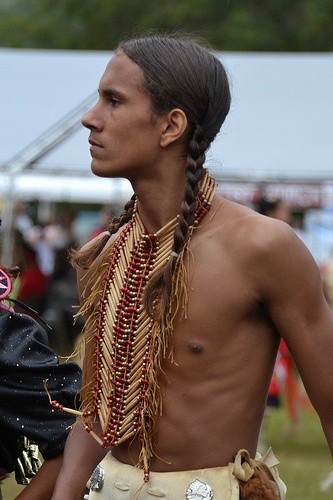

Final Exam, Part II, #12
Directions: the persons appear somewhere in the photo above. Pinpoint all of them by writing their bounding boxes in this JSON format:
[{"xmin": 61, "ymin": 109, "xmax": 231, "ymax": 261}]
[
  {"xmin": 50, "ymin": 32, "xmax": 332, "ymax": 500},
  {"xmin": 1, "ymin": 219, "xmax": 85, "ymax": 500}
]
